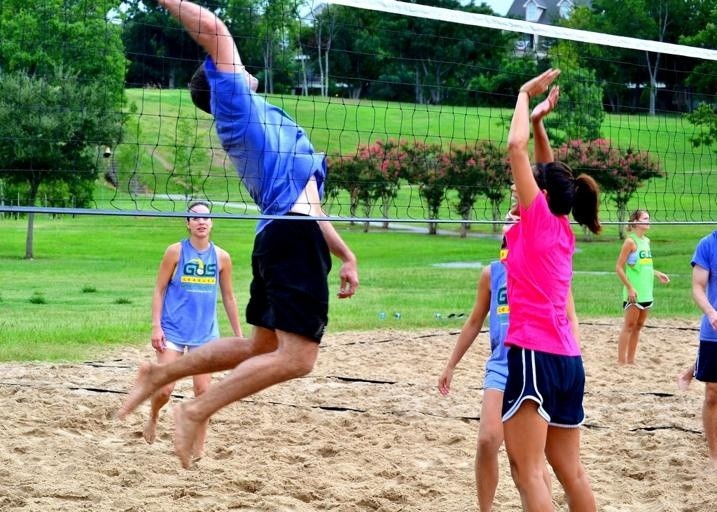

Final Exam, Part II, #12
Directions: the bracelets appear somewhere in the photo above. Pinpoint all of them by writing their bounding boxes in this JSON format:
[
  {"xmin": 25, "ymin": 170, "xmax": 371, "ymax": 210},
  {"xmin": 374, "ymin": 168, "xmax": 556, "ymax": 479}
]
[{"xmin": 518, "ymin": 89, "xmax": 530, "ymax": 99}]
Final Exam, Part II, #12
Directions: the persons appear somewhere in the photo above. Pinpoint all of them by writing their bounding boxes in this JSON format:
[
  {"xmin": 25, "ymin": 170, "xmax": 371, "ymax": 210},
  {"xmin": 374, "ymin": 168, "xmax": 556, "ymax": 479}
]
[
  {"xmin": 690, "ymin": 230, "xmax": 717, "ymax": 460},
  {"xmin": 119, "ymin": 0, "xmax": 359, "ymax": 470},
  {"xmin": 142, "ymin": 202, "xmax": 243, "ymax": 457},
  {"xmin": 615, "ymin": 210, "xmax": 669, "ymax": 365},
  {"xmin": 437, "ymin": 205, "xmax": 579, "ymax": 512},
  {"xmin": 501, "ymin": 68, "xmax": 601, "ymax": 512}
]
